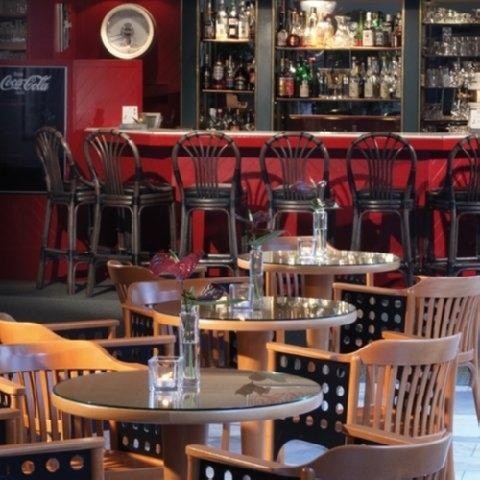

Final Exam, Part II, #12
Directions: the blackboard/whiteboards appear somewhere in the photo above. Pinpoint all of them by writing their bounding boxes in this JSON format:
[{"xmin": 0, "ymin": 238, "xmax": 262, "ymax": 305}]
[{"xmin": 0, "ymin": 64, "xmax": 68, "ymax": 195}]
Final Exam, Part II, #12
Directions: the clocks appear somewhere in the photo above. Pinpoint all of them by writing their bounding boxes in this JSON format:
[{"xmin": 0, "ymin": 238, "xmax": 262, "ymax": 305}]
[{"xmin": 99, "ymin": 3, "xmax": 157, "ymax": 62}]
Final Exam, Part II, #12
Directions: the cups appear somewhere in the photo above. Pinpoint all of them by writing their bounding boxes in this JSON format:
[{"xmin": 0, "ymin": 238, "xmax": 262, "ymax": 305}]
[
  {"xmin": 229, "ymin": 283, "xmax": 255, "ymax": 313},
  {"xmin": 422, "ymin": 6, "xmax": 480, "ymax": 24},
  {"xmin": 297, "ymin": 236, "xmax": 317, "ymax": 259},
  {"xmin": 423, "ymin": 27, "xmax": 480, "ymax": 57},
  {"xmin": 147, "ymin": 355, "xmax": 183, "ymax": 397},
  {"xmin": 420, "ymin": 58, "xmax": 480, "ymax": 90},
  {"xmin": 421, "ymin": 92, "xmax": 470, "ymax": 120},
  {"xmin": 467, "ymin": 102, "xmax": 480, "ymax": 134}
]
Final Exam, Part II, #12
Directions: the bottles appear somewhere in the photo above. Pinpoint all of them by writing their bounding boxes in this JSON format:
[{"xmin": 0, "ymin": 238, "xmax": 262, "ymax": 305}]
[
  {"xmin": 203, "ymin": 1, "xmax": 255, "ymax": 39},
  {"xmin": 200, "ymin": 52, "xmax": 255, "ymax": 91},
  {"xmin": 277, "ymin": 6, "xmax": 401, "ymax": 47},
  {"xmin": 277, "ymin": 55, "xmax": 401, "ymax": 99},
  {"xmin": 202, "ymin": 107, "xmax": 255, "ymax": 131}
]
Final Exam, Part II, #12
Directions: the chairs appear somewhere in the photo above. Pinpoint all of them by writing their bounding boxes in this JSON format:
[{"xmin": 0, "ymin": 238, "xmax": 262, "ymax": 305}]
[
  {"xmin": 346, "ymin": 131, "xmax": 419, "ymax": 287},
  {"xmin": 171, "ymin": 130, "xmax": 247, "ymax": 276},
  {"xmin": 259, "ymin": 131, "xmax": 337, "ymax": 247},
  {"xmin": 32, "ymin": 127, "xmax": 126, "ymax": 294},
  {"xmin": 83, "ymin": 128, "xmax": 176, "ymax": 297},
  {"xmin": 423, "ymin": 134, "xmax": 480, "ymax": 278}
]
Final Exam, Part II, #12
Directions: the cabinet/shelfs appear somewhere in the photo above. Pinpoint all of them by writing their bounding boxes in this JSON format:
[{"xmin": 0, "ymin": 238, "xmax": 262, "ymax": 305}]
[
  {"xmin": 257, "ymin": 0, "xmax": 403, "ymax": 130},
  {"xmin": 405, "ymin": 0, "xmax": 480, "ymax": 132},
  {"xmin": 179, "ymin": 0, "xmax": 255, "ymax": 132}
]
